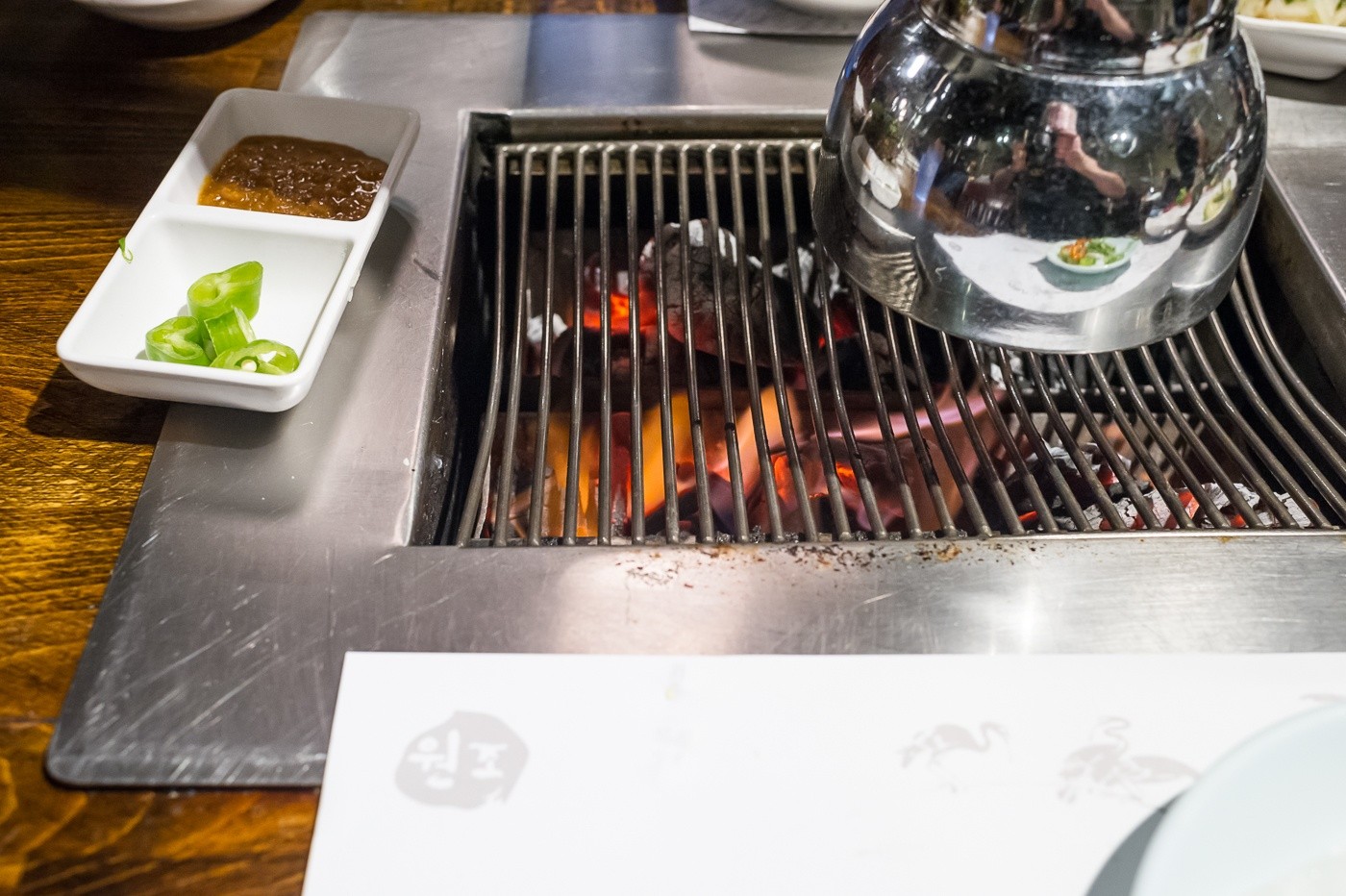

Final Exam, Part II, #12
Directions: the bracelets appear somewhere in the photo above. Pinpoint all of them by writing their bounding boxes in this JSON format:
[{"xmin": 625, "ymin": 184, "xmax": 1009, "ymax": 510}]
[{"xmin": 989, "ymin": 170, "xmax": 998, "ymax": 188}]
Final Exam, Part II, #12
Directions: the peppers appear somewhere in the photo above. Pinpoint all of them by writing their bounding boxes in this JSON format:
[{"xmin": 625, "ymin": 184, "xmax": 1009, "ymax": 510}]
[{"xmin": 142, "ymin": 260, "xmax": 301, "ymax": 375}]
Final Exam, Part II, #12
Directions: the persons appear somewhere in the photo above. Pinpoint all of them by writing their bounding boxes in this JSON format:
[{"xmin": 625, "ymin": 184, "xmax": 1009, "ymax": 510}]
[
  {"xmin": 1112, "ymin": 69, "xmax": 1263, "ymax": 228},
  {"xmin": 966, "ymin": 132, "xmax": 1127, "ymax": 240},
  {"xmin": 988, "ymin": 0, "xmax": 1149, "ymax": 59}
]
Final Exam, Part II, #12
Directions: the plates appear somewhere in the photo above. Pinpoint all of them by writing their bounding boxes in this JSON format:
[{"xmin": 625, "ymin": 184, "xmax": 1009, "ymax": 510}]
[
  {"xmin": 783, "ymin": 1, "xmax": 884, "ymax": 19},
  {"xmin": 80, "ymin": 0, "xmax": 273, "ymax": 28},
  {"xmin": 57, "ymin": 89, "xmax": 419, "ymax": 413},
  {"xmin": 1237, "ymin": 14, "xmax": 1346, "ymax": 80}
]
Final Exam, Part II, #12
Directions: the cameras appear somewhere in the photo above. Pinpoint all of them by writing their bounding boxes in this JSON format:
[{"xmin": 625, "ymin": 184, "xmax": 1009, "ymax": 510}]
[{"xmin": 1024, "ymin": 125, "xmax": 1061, "ymax": 165}]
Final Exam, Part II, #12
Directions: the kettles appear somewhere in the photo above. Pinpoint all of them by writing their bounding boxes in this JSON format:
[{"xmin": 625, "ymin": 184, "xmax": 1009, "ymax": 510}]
[{"xmin": 811, "ymin": 0, "xmax": 1267, "ymax": 357}]
